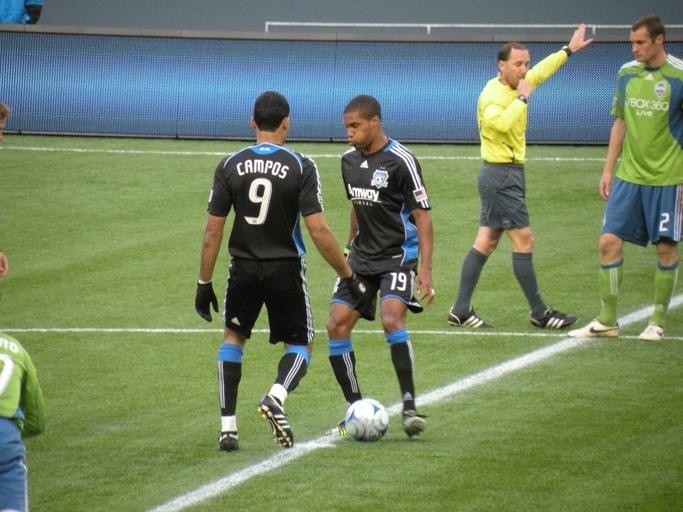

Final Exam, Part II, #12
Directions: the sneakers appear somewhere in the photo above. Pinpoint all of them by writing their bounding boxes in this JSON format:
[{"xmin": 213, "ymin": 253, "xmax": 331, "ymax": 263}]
[
  {"xmin": 636, "ymin": 323, "xmax": 669, "ymax": 345},
  {"xmin": 566, "ymin": 319, "xmax": 623, "ymax": 341},
  {"xmin": 528, "ymin": 308, "xmax": 579, "ymax": 330},
  {"xmin": 445, "ymin": 303, "xmax": 495, "ymax": 330},
  {"xmin": 258, "ymin": 388, "xmax": 301, "ymax": 450},
  {"xmin": 400, "ymin": 410, "xmax": 425, "ymax": 437},
  {"xmin": 213, "ymin": 426, "xmax": 243, "ymax": 451}
]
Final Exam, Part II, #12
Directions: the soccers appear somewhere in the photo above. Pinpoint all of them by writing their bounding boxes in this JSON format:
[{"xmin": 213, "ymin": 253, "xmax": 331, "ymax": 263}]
[{"xmin": 346, "ymin": 398, "xmax": 388, "ymax": 440}]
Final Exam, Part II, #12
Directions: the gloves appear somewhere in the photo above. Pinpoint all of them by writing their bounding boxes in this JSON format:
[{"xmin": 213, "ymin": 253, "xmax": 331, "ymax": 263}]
[
  {"xmin": 344, "ymin": 271, "xmax": 370, "ymax": 311},
  {"xmin": 193, "ymin": 280, "xmax": 220, "ymax": 323}
]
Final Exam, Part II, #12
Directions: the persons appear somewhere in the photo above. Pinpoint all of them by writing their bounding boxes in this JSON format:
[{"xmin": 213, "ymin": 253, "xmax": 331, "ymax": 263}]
[
  {"xmin": 195, "ymin": 91, "xmax": 370, "ymax": 453},
  {"xmin": 0, "ymin": 250, "xmax": 9, "ymax": 279},
  {"xmin": 567, "ymin": 14, "xmax": 683, "ymax": 340},
  {"xmin": 0, "ymin": 0, "xmax": 44, "ymax": 25},
  {"xmin": 326, "ymin": 95, "xmax": 437, "ymax": 436},
  {"xmin": 445, "ymin": 20, "xmax": 579, "ymax": 331},
  {"xmin": 0, "ymin": 333, "xmax": 48, "ymax": 512},
  {"xmin": 0, "ymin": 102, "xmax": 9, "ymax": 141}
]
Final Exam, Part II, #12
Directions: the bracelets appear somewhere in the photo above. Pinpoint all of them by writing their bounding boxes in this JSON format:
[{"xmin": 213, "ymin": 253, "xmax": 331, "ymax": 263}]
[{"xmin": 562, "ymin": 46, "xmax": 573, "ymax": 57}]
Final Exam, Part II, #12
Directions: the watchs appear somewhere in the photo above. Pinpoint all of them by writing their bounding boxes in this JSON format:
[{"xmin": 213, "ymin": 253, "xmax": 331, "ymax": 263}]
[{"xmin": 516, "ymin": 95, "xmax": 528, "ymax": 104}]
